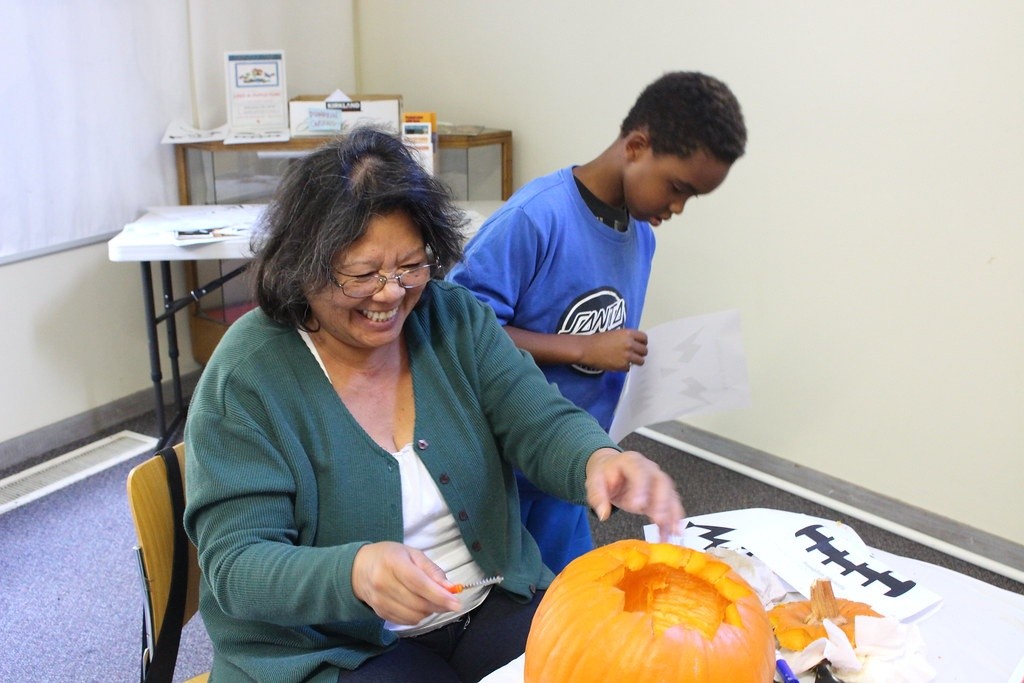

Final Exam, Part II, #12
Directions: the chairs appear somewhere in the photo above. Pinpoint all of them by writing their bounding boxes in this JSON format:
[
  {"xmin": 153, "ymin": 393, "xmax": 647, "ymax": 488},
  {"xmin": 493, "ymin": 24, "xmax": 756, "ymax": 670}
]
[{"xmin": 126, "ymin": 449, "xmax": 219, "ymax": 683}]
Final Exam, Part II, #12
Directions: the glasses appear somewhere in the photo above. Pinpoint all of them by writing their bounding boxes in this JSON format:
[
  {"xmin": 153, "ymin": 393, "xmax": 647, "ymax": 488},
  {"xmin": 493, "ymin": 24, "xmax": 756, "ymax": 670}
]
[{"xmin": 325, "ymin": 240, "xmax": 440, "ymax": 299}]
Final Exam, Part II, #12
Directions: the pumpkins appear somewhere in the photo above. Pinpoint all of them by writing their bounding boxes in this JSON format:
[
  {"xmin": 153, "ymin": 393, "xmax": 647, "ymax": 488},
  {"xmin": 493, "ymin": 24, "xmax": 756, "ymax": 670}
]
[{"xmin": 523, "ymin": 539, "xmax": 883, "ymax": 682}]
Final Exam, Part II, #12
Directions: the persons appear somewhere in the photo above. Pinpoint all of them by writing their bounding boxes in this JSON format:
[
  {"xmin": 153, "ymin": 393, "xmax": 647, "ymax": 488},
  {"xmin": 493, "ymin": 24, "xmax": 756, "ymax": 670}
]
[
  {"xmin": 429, "ymin": 67, "xmax": 750, "ymax": 581},
  {"xmin": 183, "ymin": 120, "xmax": 690, "ymax": 683}
]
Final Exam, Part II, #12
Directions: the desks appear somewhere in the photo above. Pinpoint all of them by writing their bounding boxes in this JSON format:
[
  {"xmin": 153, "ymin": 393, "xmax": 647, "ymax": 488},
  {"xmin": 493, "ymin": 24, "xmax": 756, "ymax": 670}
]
[
  {"xmin": 169, "ymin": 119, "xmax": 518, "ymax": 372},
  {"xmin": 574, "ymin": 412, "xmax": 1024, "ymax": 682}
]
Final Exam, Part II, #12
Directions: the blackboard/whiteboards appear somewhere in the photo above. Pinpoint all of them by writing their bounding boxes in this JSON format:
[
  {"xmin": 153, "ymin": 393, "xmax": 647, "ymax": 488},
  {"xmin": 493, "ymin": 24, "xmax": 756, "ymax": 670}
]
[{"xmin": 0, "ymin": 0, "xmax": 199, "ymax": 265}]
